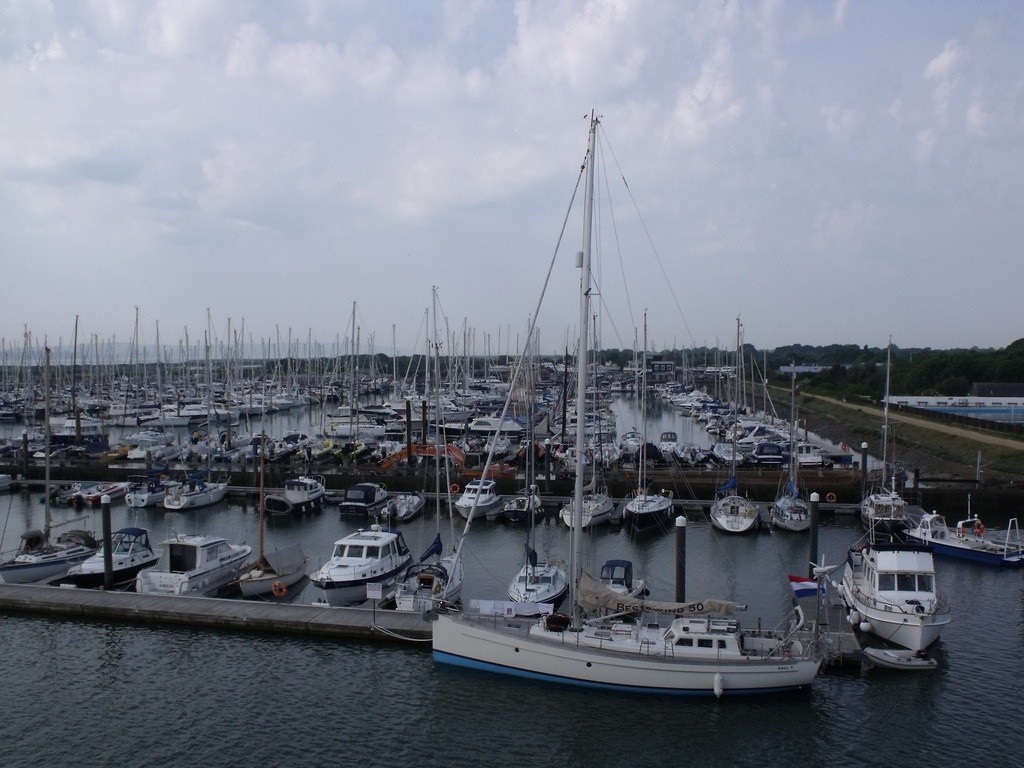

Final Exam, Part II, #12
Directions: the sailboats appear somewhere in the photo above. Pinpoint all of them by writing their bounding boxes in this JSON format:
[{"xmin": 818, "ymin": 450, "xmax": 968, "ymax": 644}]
[
  {"xmin": 0, "ymin": 285, "xmax": 858, "ymax": 537},
  {"xmin": 395, "ymin": 288, "xmax": 463, "ymax": 612},
  {"xmin": 431, "ymin": 109, "xmax": 823, "ymax": 697},
  {"xmin": 709, "ymin": 313, "xmax": 760, "ymax": 532},
  {"xmin": 0, "ymin": 346, "xmax": 102, "ymax": 584},
  {"xmin": 621, "ymin": 308, "xmax": 675, "ymax": 532},
  {"xmin": 507, "ymin": 310, "xmax": 568, "ymax": 603},
  {"xmin": 237, "ymin": 429, "xmax": 308, "ymax": 595},
  {"xmin": 859, "ymin": 346, "xmax": 909, "ymax": 531},
  {"xmin": 770, "ymin": 358, "xmax": 811, "ymax": 531},
  {"xmin": 560, "ymin": 314, "xmax": 614, "ymax": 528}
]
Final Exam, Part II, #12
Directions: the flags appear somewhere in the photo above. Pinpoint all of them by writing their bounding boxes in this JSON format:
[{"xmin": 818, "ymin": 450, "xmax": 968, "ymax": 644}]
[{"xmin": 789, "ymin": 575, "xmax": 826, "ymax": 599}]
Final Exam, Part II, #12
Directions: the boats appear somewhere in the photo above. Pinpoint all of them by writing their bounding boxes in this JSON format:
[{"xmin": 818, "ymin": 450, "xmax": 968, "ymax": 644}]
[
  {"xmin": 824, "ymin": 519, "xmax": 952, "ymax": 652},
  {"xmin": 903, "ymin": 510, "xmax": 1024, "ymax": 564},
  {"xmin": 47, "ymin": 527, "xmax": 164, "ymax": 590},
  {"xmin": 585, "ymin": 560, "xmax": 644, "ymax": 617},
  {"xmin": 136, "ymin": 527, "xmax": 252, "ymax": 598},
  {"xmin": 309, "ymin": 524, "xmax": 410, "ymax": 590}
]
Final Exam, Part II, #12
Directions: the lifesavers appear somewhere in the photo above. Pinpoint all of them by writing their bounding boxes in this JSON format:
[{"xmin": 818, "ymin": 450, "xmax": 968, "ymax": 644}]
[
  {"xmin": 272, "ymin": 580, "xmax": 287, "ymax": 598},
  {"xmin": 972, "ymin": 521, "xmax": 986, "ymax": 537},
  {"xmin": 826, "ymin": 492, "xmax": 837, "ymax": 503},
  {"xmin": 450, "ymin": 483, "xmax": 459, "ymax": 493}
]
